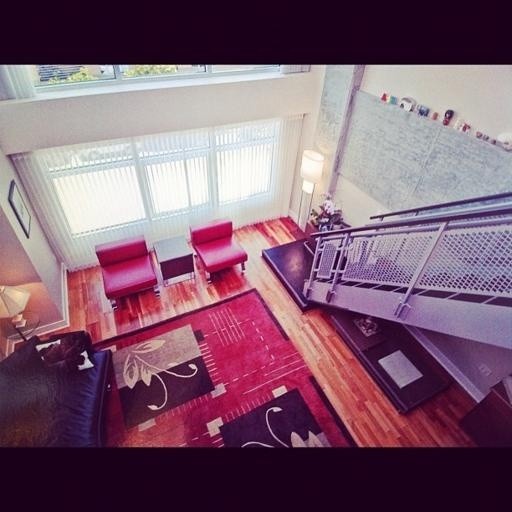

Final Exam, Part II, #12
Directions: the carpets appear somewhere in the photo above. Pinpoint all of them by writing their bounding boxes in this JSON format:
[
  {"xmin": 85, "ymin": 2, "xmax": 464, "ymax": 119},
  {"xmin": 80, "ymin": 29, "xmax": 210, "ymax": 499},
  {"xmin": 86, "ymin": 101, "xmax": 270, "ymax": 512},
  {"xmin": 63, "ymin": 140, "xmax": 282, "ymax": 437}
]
[{"xmin": 89, "ymin": 288, "xmax": 360, "ymax": 447}]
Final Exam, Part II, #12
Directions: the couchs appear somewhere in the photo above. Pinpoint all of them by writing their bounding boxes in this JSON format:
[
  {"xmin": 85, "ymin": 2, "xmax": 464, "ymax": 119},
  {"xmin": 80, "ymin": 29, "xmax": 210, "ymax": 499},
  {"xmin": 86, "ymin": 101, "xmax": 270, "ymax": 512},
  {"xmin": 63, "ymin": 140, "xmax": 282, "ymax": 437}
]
[{"xmin": 0, "ymin": 331, "xmax": 110, "ymax": 447}]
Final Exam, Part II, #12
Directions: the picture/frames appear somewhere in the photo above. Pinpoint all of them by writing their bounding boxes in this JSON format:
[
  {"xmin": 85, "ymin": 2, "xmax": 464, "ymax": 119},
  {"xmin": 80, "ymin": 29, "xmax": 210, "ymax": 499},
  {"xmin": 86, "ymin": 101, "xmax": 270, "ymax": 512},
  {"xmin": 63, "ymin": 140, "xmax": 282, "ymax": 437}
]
[{"xmin": 8, "ymin": 179, "xmax": 32, "ymax": 239}]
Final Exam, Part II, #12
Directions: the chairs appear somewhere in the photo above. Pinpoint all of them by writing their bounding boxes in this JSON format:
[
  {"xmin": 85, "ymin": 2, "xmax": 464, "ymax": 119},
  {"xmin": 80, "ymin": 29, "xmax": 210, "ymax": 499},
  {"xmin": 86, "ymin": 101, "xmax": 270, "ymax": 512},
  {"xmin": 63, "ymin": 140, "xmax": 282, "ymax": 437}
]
[
  {"xmin": 190, "ymin": 219, "xmax": 248, "ymax": 284},
  {"xmin": 94, "ymin": 235, "xmax": 162, "ymax": 310}
]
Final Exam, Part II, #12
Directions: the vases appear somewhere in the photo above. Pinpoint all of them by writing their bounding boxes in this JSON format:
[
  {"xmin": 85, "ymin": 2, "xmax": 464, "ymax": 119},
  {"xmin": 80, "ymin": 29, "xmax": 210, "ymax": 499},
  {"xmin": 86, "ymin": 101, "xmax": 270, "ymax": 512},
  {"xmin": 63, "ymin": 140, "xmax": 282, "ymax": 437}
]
[{"xmin": 332, "ymin": 223, "xmax": 342, "ymax": 231}]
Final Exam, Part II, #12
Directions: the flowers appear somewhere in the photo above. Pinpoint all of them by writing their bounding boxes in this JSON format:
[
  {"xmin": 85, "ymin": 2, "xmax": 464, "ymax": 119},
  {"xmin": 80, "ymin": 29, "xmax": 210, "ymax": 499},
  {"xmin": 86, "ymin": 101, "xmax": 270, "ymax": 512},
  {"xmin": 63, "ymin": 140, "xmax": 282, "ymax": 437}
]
[{"xmin": 308, "ymin": 191, "xmax": 343, "ymax": 232}]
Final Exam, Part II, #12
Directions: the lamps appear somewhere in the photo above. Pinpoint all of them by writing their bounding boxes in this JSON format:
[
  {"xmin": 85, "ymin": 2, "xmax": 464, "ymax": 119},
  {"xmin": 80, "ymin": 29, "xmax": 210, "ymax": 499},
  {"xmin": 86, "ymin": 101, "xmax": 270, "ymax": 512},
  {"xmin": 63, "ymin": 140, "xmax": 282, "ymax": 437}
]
[
  {"xmin": 0, "ymin": 284, "xmax": 31, "ymax": 343},
  {"xmin": 299, "ymin": 150, "xmax": 326, "ymax": 193}
]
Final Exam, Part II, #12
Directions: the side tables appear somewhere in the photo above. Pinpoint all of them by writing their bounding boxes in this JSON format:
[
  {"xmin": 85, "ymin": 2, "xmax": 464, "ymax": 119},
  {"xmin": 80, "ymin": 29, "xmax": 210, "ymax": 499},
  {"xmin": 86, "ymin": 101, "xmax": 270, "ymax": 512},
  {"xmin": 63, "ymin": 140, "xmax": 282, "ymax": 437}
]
[{"xmin": 152, "ymin": 235, "xmax": 195, "ymax": 287}]
